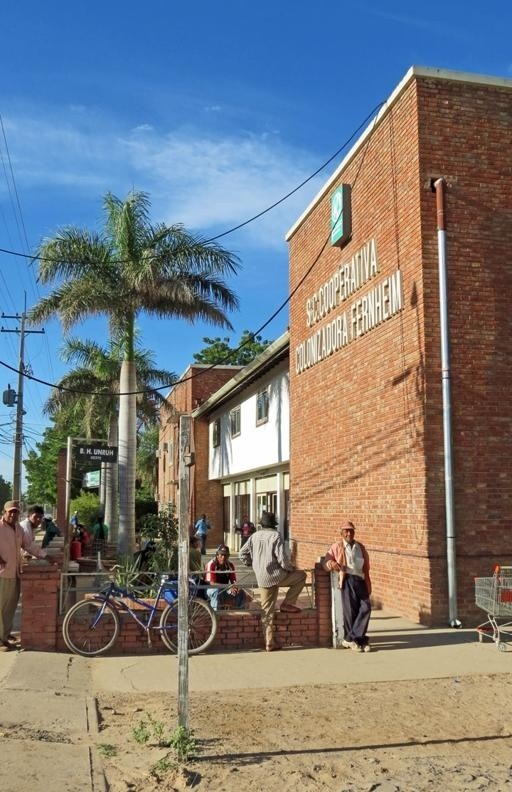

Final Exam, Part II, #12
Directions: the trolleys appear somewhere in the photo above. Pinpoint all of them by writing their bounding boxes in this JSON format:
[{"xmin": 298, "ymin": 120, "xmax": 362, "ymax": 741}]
[{"xmin": 472, "ymin": 564, "xmax": 512, "ymax": 652}]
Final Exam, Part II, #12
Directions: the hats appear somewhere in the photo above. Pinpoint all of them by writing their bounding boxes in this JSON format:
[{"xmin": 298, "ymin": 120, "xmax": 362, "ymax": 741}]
[
  {"xmin": 340, "ymin": 521, "xmax": 356, "ymax": 529},
  {"xmin": 217, "ymin": 544, "xmax": 230, "ymax": 555},
  {"xmin": 4, "ymin": 501, "xmax": 21, "ymax": 513},
  {"xmin": 260, "ymin": 512, "xmax": 278, "ymax": 527}
]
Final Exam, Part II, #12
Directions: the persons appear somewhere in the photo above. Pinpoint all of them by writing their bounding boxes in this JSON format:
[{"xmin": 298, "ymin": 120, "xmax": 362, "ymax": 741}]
[
  {"xmin": 0, "ymin": 500, "xmax": 64, "ymax": 652},
  {"xmin": 194, "ymin": 513, "xmax": 212, "ymax": 554},
  {"xmin": 167, "ymin": 537, "xmax": 209, "ymax": 601},
  {"xmin": 202, "ymin": 543, "xmax": 247, "ymax": 612},
  {"xmin": 18, "ymin": 504, "xmax": 45, "ymax": 559},
  {"xmin": 131, "ymin": 540, "xmax": 158, "ymax": 594},
  {"xmin": 38, "ymin": 510, "xmax": 110, "ymax": 558},
  {"xmin": 238, "ymin": 511, "xmax": 308, "ymax": 651},
  {"xmin": 233, "ymin": 515, "xmax": 256, "ymax": 547},
  {"xmin": 322, "ymin": 521, "xmax": 373, "ymax": 653}
]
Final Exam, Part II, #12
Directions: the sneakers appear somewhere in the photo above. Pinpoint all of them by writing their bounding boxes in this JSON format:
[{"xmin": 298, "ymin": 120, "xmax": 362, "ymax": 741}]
[
  {"xmin": 362, "ymin": 642, "xmax": 372, "ymax": 652},
  {"xmin": 7, "ymin": 635, "xmax": 16, "ymax": 642},
  {"xmin": 266, "ymin": 643, "xmax": 282, "ymax": 652},
  {"xmin": 341, "ymin": 638, "xmax": 364, "ymax": 652},
  {"xmin": 0, "ymin": 642, "xmax": 17, "ymax": 652},
  {"xmin": 280, "ymin": 604, "xmax": 301, "ymax": 613}
]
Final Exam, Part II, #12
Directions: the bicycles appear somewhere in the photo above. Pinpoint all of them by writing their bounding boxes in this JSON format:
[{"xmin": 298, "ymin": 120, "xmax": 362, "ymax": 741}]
[{"xmin": 63, "ymin": 551, "xmax": 219, "ymax": 658}]
